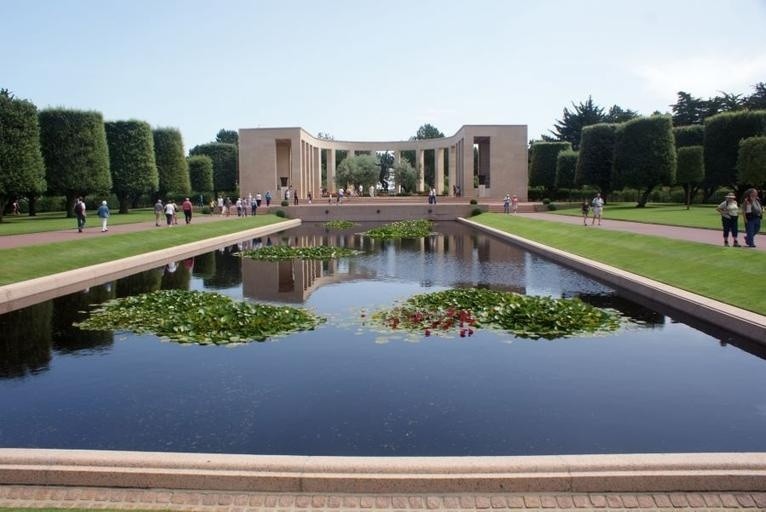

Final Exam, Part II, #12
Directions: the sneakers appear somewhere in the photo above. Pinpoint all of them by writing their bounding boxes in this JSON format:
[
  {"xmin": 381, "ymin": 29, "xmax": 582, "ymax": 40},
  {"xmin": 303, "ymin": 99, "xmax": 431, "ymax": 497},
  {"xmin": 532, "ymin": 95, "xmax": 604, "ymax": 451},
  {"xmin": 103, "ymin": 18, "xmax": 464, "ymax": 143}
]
[{"xmin": 745, "ymin": 237, "xmax": 755, "ymax": 247}]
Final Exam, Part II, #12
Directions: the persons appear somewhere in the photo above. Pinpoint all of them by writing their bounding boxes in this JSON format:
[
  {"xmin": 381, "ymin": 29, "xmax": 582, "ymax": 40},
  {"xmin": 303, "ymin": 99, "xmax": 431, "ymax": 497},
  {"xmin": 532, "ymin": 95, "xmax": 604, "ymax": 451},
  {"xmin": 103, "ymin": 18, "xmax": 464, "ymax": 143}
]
[
  {"xmin": 581, "ymin": 199, "xmax": 590, "ymax": 226},
  {"xmin": 73, "ymin": 196, "xmax": 87, "ymax": 232},
  {"xmin": 742, "ymin": 188, "xmax": 762, "ymax": 247},
  {"xmin": 154, "ymin": 183, "xmax": 375, "ymax": 228},
  {"xmin": 591, "ymin": 193, "xmax": 605, "ymax": 226},
  {"xmin": 453, "ymin": 185, "xmax": 456, "ymax": 196},
  {"xmin": 158, "ymin": 257, "xmax": 194, "ymax": 277},
  {"xmin": 219, "ymin": 236, "xmax": 272, "ymax": 254},
  {"xmin": 10, "ymin": 200, "xmax": 21, "ymax": 215},
  {"xmin": 375, "ymin": 157, "xmax": 394, "ymax": 191},
  {"xmin": 82, "ymin": 288, "xmax": 89, "ymax": 294},
  {"xmin": 96, "ymin": 200, "xmax": 110, "ymax": 232},
  {"xmin": 503, "ymin": 193, "xmax": 511, "ymax": 214},
  {"xmin": 103, "ymin": 282, "xmax": 112, "ymax": 292},
  {"xmin": 432, "ymin": 188, "xmax": 437, "ymax": 204},
  {"xmin": 428, "ymin": 190, "xmax": 433, "ymax": 203},
  {"xmin": 456, "ymin": 186, "xmax": 459, "ymax": 198},
  {"xmin": 716, "ymin": 193, "xmax": 741, "ymax": 247},
  {"xmin": 510, "ymin": 195, "xmax": 519, "ymax": 215}
]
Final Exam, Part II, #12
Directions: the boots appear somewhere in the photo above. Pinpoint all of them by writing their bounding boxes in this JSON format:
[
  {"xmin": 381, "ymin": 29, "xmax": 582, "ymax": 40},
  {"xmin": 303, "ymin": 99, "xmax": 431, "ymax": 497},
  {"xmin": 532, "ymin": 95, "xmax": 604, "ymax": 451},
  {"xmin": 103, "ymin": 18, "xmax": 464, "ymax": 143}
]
[
  {"xmin": 733, "ymin": 240, "xmax": 741, "ymax": 247},
  {"xmin": 725, "ymin": 241, "xmax": 730, "ymax": 247}
]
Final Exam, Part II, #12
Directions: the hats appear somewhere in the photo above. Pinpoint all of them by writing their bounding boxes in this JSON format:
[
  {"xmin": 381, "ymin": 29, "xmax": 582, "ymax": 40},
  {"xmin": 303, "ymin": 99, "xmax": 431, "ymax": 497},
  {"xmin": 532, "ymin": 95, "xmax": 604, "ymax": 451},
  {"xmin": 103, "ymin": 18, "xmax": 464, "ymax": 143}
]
[{"xmin": 725, "ymin": 192, "xmax": 736, "ymax": 199}]
